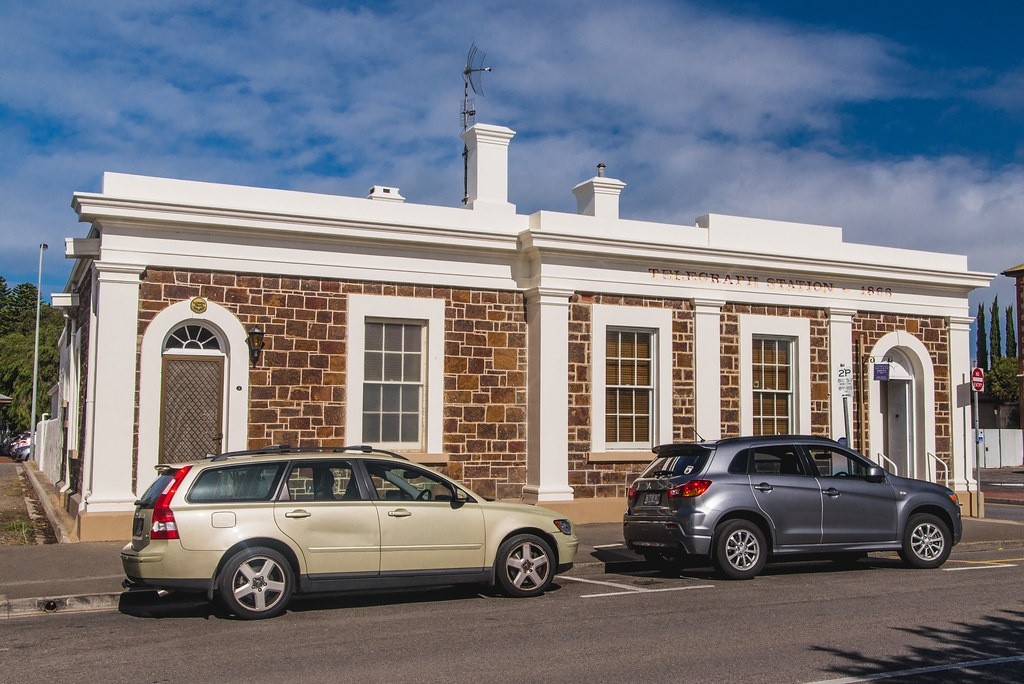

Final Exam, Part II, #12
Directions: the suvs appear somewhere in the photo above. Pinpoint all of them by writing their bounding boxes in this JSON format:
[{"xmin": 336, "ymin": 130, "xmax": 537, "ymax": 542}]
[
  {"xmin": 119, "ymin": 447, "xmax": 581, "ymax": 620},
  {"xmin": 623, "ymin": 433, "xmax": 966, "ymax": 582}
]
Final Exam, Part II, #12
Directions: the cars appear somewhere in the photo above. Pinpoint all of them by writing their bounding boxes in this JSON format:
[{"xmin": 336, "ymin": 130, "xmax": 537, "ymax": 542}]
[{"xmin": 0, "ymin": 431, "xmax": 37, "ymax": 460}]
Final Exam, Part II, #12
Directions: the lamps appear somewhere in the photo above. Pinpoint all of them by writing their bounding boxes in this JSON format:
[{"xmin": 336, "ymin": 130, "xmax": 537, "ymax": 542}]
[{"xmin": 246, "ymin": 326, "xmax": 265, "ymax": 370}]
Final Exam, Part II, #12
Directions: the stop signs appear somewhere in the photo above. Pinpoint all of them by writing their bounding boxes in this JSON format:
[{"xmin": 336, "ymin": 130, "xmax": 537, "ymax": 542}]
[{"xmin": 971, "ymin": 367, "xmax": 984, "ymax": 392}]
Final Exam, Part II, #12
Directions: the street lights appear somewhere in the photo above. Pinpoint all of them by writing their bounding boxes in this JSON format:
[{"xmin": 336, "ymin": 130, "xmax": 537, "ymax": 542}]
[{"xmin": 29, "ymin": 242, "xmax": 48, "ymax": 461}]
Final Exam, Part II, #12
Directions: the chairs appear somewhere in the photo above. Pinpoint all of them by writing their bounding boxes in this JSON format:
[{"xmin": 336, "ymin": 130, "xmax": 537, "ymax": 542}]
[
  {"xmin": 316, "ymin": 470, "xmax": 336, "ymax": 500},
  {"xmin": 779, "ymin": 454, "xmax": 798, "ymax": 474}
]
